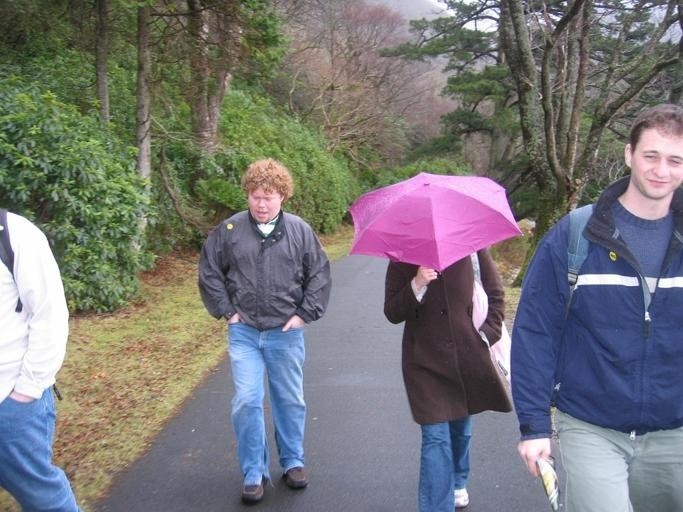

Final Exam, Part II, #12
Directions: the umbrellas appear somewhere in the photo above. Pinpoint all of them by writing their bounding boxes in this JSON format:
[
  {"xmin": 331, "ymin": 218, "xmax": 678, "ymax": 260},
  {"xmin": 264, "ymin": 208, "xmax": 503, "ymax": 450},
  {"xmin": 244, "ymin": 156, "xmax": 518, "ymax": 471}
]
[{"xmin": 346, "ymin": 171, "xmax": 524, "ymax": 273}]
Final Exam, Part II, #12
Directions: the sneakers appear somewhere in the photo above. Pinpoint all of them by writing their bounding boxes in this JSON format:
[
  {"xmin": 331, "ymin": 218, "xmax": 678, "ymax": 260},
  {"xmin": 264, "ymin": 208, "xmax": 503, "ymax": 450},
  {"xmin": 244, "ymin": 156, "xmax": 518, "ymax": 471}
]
[
  {"xmin": 454, "ymin": 488, "xmax": 469, "ymax": 508},
  {"xmin": 284, "ymin": 467, "xmax": 308, "ymax": 488},
  {"xmin": 241, "ymin": 476, "xmax": 264, "ymax": 501}
]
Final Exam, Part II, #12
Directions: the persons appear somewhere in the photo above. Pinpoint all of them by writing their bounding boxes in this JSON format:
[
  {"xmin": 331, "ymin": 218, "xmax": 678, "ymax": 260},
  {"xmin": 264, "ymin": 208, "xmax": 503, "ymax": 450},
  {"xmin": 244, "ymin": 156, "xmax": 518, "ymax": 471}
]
[
  {"xmin": 195, "ymin": 158, "xmax": 331, "ymax": 505},
  {"xmin": 382, "ymin": 244, "xmax": 504, "ymax": 512},
  {"xmin": 0, "ymin": 207, "xmax": 79, "ymax": 512},
  {"xmin": 510, "ymin": 101, "xmax": 681, "ymax": 512}
]
